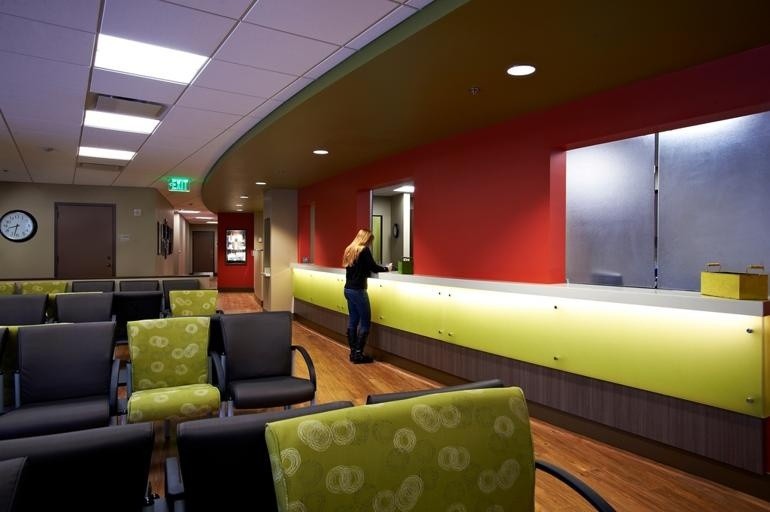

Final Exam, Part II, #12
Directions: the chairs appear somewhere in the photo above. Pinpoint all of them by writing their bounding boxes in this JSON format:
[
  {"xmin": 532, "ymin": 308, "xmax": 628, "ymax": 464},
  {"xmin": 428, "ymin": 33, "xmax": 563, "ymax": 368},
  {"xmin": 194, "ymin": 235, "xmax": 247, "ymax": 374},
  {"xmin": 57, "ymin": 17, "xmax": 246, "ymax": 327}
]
[
  {"xmin": 0, "ymin": 274, "xmax": 318, "ymax": 373},
  {"xmin": 367, "ymin": 380, "xmax": 505, "ymax": 406},
  {"xmin": 265, "ymin": 387, "xmax": 616, "ymax": 510},
  {"xmin": 165, "ymin": 401, "xmax": 354, "ymax": 512},
  {"xmin": 3, "ymin": 421, "xmax": 155, "ymax": 511}
]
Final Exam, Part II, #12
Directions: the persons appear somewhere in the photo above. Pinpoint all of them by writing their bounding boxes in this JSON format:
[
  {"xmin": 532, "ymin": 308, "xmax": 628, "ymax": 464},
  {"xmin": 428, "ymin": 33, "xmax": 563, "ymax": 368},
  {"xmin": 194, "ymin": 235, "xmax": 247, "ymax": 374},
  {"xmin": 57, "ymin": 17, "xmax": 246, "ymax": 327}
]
[{"xmin": 341, "ymin": 228, "xmax": 394, "ymax": 365}]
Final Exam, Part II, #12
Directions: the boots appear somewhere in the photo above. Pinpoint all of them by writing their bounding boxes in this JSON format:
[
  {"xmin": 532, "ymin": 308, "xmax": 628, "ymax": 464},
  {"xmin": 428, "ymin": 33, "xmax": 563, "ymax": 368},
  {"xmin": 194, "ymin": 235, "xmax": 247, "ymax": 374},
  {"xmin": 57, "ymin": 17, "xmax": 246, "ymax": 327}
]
[{"xmin": 346, "ymin": 326, "xmax": 375, "ymax": 364}]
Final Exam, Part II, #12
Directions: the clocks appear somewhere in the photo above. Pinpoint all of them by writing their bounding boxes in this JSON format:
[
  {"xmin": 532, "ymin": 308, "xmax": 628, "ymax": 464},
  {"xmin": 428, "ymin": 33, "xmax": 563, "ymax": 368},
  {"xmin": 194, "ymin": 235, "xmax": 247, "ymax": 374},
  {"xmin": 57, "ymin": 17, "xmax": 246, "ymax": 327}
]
[{"xmin": 0, "ymin": 209, "xmax": 39, "ymax": 244}]
